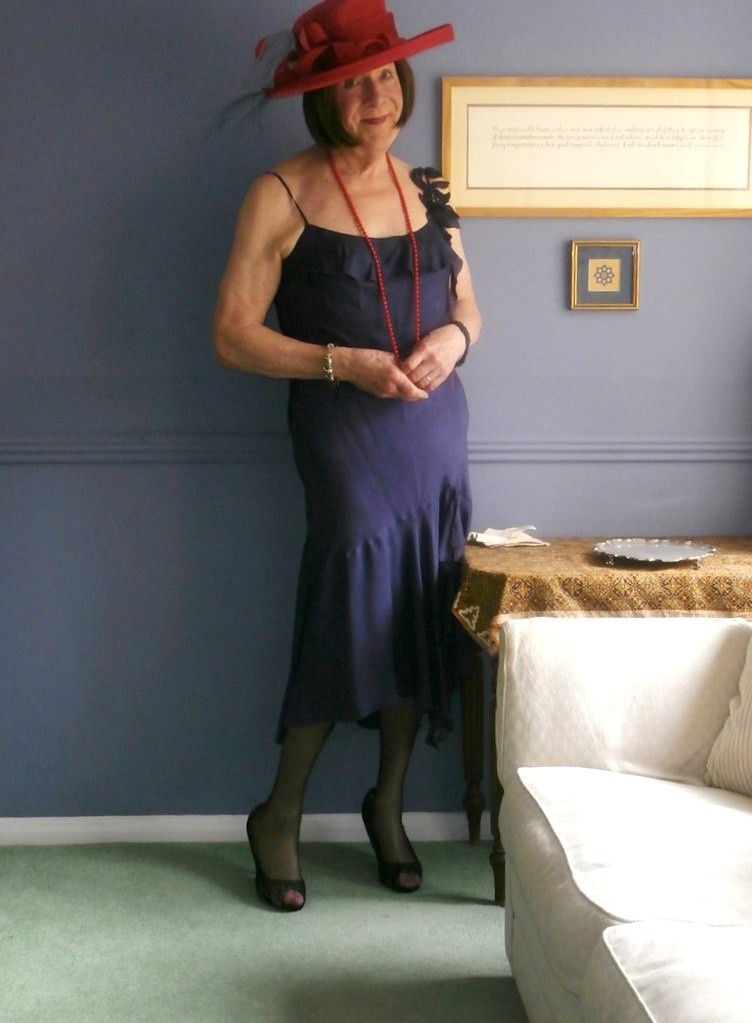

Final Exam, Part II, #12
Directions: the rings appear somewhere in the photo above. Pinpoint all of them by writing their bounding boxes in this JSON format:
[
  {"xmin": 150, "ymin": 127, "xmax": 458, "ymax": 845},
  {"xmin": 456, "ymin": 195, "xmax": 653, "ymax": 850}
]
[{"xmin": 427, "ymin": 376, "xmax": 432, "ymax": 383}]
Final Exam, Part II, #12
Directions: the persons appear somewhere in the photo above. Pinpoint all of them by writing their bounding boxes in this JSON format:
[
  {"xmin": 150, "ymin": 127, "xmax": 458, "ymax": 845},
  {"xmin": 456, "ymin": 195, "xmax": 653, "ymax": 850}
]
[{"xmin": 213, "ymin": 0, "xmax": 482, "ymax": 913}]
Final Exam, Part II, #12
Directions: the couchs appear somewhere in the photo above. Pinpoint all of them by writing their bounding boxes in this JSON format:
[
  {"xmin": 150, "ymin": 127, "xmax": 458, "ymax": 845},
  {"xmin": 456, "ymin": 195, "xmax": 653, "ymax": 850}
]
[{"xmin": 492, "ymin": 609, "xmax": 752, "ymax": 1023}]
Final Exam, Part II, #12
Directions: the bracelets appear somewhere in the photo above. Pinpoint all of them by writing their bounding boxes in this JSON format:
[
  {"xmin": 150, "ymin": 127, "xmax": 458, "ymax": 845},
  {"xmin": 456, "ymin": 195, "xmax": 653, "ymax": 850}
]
[
  {"xmin": 323, "ymin": 342, "xmax": 337, "ymax": 385},
  {"xmin": 443, "ymin": 320, "xmax": 471, "ymax": 367}
]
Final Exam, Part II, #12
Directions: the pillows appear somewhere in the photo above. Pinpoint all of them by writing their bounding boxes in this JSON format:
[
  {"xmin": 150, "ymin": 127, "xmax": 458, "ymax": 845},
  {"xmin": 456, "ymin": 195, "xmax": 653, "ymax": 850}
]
[{"xmin": 700, "ymin": 626, "xmax": 749, "ymax": 803}]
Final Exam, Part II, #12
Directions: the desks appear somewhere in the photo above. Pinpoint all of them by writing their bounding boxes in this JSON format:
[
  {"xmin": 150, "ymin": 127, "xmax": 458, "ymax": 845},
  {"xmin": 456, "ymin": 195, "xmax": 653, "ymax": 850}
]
[{"xmin": 456, "ymin": 532, "xmax": 751, "ymax": 904}]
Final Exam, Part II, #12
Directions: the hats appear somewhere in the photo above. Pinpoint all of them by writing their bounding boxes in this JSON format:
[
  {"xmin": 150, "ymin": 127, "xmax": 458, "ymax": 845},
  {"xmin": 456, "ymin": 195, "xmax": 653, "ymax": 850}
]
[{"xmin": 255, "ymin": 1, "xmax": 456, "ymax": 99}]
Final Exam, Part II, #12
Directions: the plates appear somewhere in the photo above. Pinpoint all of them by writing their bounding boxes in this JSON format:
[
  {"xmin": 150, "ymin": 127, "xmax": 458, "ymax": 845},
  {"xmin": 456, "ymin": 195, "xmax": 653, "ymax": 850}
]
[{"xmin": 592, "ymin": 537, "xmax": 717, "ymax": 570}]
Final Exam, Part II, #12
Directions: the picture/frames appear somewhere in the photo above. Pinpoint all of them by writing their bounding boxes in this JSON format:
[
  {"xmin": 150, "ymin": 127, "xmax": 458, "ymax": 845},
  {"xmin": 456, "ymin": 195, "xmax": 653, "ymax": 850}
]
[
  {"xmin": 571, "ymin": 238, "xmax": 639, "ymax": 312},
  {"xmin": 442, "ymin": 75, "xmax": 751, "ymax": 220}
]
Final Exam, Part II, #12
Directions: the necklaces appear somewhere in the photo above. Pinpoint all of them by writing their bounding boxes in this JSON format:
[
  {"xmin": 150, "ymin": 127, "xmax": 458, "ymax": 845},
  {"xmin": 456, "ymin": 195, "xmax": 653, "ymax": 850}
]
[{"xmin": 325, "ymin": 143, "xmax": 420, "ymax": 370}]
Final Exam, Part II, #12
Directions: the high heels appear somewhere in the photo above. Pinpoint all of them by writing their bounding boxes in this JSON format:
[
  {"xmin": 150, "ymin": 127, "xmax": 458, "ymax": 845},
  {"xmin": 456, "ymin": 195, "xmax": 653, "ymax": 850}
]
[
  {"xmin": 359, "ymin": 787, "xmax": 423, "ymax": 894},
  {"xmin": 247, "ymin": 801, "xmax": 307, "ymax": 912}
]
